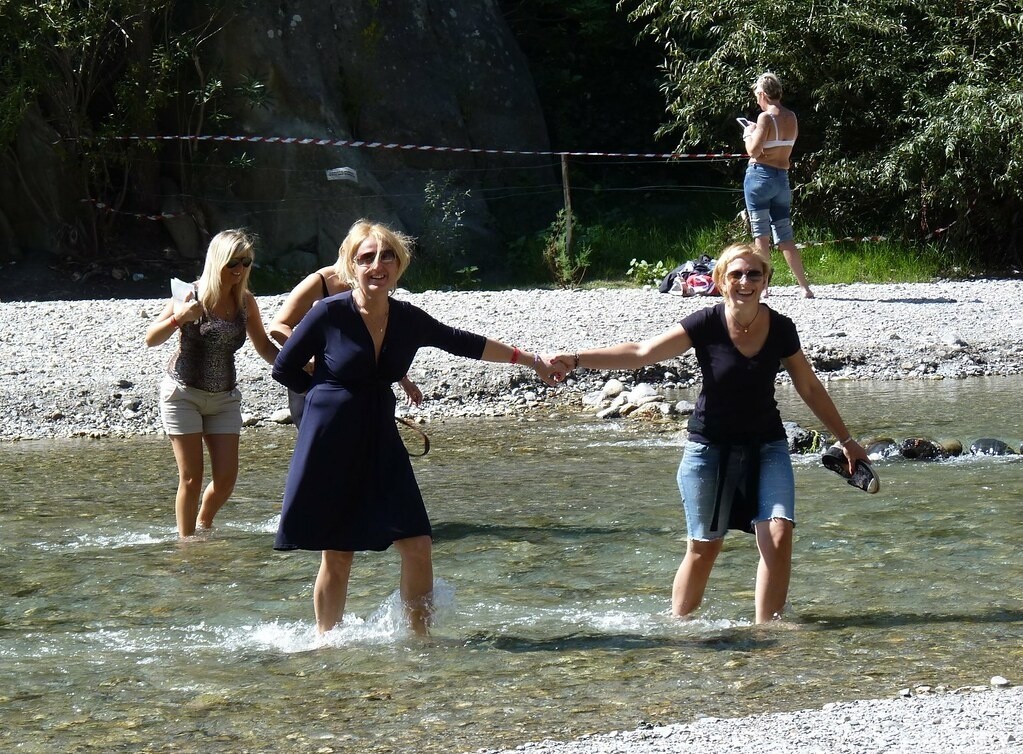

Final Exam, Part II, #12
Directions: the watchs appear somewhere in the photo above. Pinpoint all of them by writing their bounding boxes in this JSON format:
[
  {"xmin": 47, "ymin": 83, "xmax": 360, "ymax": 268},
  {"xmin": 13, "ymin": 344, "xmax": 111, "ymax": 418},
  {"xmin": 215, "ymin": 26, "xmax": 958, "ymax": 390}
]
[{"xmin": 531, "ymin": 353, "xmax": 540, "ymax": 370}]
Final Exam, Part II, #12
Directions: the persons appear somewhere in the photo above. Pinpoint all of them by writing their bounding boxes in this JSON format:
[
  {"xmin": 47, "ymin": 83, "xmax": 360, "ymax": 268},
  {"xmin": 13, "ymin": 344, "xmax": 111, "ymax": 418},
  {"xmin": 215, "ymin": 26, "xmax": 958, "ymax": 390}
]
[
  {"xmin": 271, "ymin": 217, "xmax": 567, "ymax": 638},
  {"xmin": 550, "ymin": 242, "xmax": 871, "ymax": 625},
  {"xmin": 146, "ymin": 228, "xmax": 314, "ymax": 539},
  {"xmin": 743, "ymin": 72, "xmax": 814, "ymax": 298}
]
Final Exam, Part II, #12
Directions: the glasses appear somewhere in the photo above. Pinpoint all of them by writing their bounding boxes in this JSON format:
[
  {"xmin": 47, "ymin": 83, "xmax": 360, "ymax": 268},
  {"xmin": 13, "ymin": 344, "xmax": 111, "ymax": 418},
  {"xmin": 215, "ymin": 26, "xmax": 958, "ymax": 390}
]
[
  {"xmin": 723, "ymin": 270, "xmax": 765, "ymax": 284},
  {"xmin": 351, "ymin": 249, "xmax": 399, "ymax": 266},
  {"xmin": 226, "ymin": 257, "xmax": 253, "ymax": 269}
]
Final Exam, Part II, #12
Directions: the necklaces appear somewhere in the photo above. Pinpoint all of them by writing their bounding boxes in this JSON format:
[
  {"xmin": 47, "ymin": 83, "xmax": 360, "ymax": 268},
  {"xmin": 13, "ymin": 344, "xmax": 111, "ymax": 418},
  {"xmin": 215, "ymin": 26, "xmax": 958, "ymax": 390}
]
[
  {"xmin": 361, "ymin": 303, "xmax": 389, "ymax": 332},
  {"xmin": 223, "ymin": 302, "xmax": 233, "ymax": 316},
  {"xmin": 727, "ymin": 304, "xmax": 760, "ymax": 333}
]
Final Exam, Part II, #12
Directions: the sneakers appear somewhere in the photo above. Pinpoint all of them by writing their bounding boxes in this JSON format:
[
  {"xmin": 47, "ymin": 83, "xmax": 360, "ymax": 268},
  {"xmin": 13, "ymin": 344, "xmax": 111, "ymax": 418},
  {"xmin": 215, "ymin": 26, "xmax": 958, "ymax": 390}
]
[{"xmin": 822, "ymin": 444, "xmax": 881, "ymax": 494}]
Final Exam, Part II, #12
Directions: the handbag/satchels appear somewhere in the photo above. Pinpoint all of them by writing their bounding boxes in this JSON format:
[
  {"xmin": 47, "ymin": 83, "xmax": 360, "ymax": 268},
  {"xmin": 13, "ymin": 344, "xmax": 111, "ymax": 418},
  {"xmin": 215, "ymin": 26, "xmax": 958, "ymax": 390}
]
[{"xmin": 659, "ymin": 254, "xmax": 720, "ymax": 297}]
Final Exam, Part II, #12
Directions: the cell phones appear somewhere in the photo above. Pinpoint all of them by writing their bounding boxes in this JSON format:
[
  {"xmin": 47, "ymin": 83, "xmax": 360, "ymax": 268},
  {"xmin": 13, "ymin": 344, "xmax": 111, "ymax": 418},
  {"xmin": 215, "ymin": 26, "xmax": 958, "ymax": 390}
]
[{"xmin": 735, "ymin": 118, "xmax": 751, "ymax": 131}]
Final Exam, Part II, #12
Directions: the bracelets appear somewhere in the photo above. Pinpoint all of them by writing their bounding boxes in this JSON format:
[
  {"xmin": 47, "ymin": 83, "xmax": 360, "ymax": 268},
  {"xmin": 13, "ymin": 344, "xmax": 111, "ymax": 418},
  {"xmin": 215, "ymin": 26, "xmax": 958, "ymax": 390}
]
[
  {"xmin": 744, "ymin": 135, "xmax": 751, "ymax": 141},
  {"xmin": 574, "ymin": 352, "xmax": 579, "ymax": 369},
  {"xmin": 171, "ymin": 315, "xmax": 180, "ymax": 329},
  {"xmin": 840, "ymin": 436, "xmax": 852, "ymax": 445},
  {"xmin": 303, "ymin": 362, "xmax": 312, "ymax": 373},
  {"xmin": 510, "ymin": 346, "xmax": 519, "ymax": 364}
]
